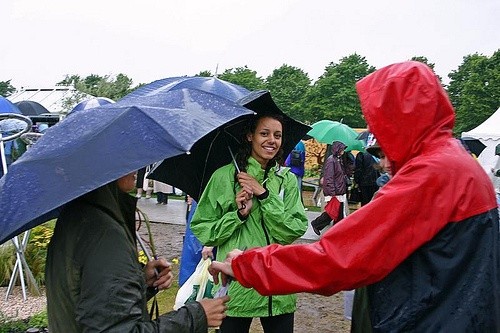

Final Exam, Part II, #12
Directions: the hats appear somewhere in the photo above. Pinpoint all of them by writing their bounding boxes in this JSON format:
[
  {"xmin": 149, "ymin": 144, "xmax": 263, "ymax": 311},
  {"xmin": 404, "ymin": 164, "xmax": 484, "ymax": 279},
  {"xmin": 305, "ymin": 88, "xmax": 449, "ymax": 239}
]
[{"xmin": 367, "ymin": 141, "xmax": 383, "ymax": 158}]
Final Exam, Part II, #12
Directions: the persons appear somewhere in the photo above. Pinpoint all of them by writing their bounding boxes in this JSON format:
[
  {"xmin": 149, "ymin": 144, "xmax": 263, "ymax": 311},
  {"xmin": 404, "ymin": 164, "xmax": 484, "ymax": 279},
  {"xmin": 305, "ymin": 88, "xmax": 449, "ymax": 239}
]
[
  {"xmin": 188, "ymin": 110, "xmax": 309, "ymax": 333},
  {"xmin": 44, "ymin": 171, "xmax": 230, "ymax": 333},
  {"xmin": 310, "ymin": 141, "xmax": 348, "ymax": 236},
  {"xmin": 349, "ymin": 148, "xmax": 378, "ymax": 208},
  {"xmin": 134, "ymin": 162, "xmax": 152, "ymax": 199},
  {"xmin": 155, "ymin": 192, "xmax": 168, "ymax": 204},
  {"xmin": 366, "ymin": 141, "xmax": 395, "ymax": 189},
  {"xmin": 1, "ymin": 131, "xmax": 19, "ymax": 167},
  {"xmin": 206, "ymin": 61, "xmax": 500, "ymax": 333},
  {"xmin": 285, "ymin": 141, "xmax": 308, "ymax": 211}
]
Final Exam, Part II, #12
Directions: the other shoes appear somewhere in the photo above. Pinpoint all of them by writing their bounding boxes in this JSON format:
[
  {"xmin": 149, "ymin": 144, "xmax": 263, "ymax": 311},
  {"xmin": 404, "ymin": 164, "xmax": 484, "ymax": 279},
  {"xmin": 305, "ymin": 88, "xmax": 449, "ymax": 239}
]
[
  {"xmin": 157, "ymin": 201, "xmax": 162, "ymax": 205},
  {"xmin": 136, "ymin": 194, "xmax": 141, "ymax": 198},
  {"xmin": 145, "ymin": 195, "xmax": 151, "ymax": 199},
  {"xmin": 310, "ymin": 220, "xmax": 320, "ymax": 236},
  {"xmin": 163, "ymin": 203, "xmax": 166, "ymax": 205}
]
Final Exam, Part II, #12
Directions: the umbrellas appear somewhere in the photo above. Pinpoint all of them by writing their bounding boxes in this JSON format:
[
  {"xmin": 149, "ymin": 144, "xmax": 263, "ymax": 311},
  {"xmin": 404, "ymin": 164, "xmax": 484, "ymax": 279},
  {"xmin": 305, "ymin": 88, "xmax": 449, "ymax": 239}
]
[
  {"xmin": 147, "ymin": 89, "xmax": 314, "ymax": 202},
  {"xmin": 306, "ymin": 120, "xmax": 366, "ymax": 154},
  {"xmin": 0, "ymin": 63, "xmax": 257, "ymax": 278},
  {"xmin": 461, "ymin": 106, "xmax": 500, "ymax": 146}
]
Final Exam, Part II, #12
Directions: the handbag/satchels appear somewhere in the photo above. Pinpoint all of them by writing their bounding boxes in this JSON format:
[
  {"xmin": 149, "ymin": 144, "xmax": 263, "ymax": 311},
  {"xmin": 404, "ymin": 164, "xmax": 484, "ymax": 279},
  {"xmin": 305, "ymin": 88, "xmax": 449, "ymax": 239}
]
[
  {"xmin": 325, "ymin": 198, "xmax": 341, "ymax": 220},
  {"xmin": 173, "ymin": 256, "xmax": 214, "ymax": 312}
]
